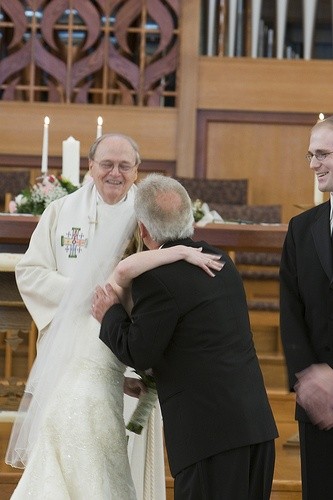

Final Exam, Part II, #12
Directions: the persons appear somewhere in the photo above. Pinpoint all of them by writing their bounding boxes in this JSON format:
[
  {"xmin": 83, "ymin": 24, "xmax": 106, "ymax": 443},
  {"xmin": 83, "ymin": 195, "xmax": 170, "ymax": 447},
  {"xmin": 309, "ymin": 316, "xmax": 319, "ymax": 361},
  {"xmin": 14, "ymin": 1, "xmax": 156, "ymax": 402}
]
[
  {"xmin": 278, "ymin": 115, "xmax": 333, "ymax": 500},
  {"xmin": 90, "ymin": 171, "xmax": 280, "ymax": 500},
  {"xmin": 4, "ymin": 197, "xmax": 224, "ymax": 500},
  {"xmin": 14, "ymin": 134, "xmax": 166, "ymax": 500}
]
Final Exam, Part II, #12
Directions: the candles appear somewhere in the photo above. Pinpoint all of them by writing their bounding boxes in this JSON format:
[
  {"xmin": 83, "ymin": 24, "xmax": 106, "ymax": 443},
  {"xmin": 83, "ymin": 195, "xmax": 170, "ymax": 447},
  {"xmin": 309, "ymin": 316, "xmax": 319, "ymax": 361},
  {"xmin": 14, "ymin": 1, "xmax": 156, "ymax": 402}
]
[
  {"xmin": 62, "ymin": 136, "xmax": 80, "ymax": 188},
  {"xmin": 41, "ymin": 116, "xmax": 50, "ymax": 173},
  {"xmin": 96, "ymin": 115, "xmax": 103, "ymax": 139}
]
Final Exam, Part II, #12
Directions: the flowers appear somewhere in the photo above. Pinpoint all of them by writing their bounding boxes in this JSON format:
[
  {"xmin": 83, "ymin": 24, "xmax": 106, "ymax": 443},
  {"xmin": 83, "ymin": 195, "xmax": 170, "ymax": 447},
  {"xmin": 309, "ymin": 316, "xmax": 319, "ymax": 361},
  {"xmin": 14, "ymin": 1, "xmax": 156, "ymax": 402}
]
[{"xmin": 9, "ymin": 175, "xmax": 79, "ymax": 215}]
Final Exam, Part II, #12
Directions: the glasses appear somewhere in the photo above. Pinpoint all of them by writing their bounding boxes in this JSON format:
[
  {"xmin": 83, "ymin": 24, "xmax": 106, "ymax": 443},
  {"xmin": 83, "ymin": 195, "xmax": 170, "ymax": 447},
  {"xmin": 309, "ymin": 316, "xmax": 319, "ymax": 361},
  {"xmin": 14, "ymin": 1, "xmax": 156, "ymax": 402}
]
[
  {"xmin": 304, "ymin": 150, "xmax": 333, "ymax": 161},
  {"xmin": 93, "ymin": 159, "xmax": 137, "ymax": 174}
]
